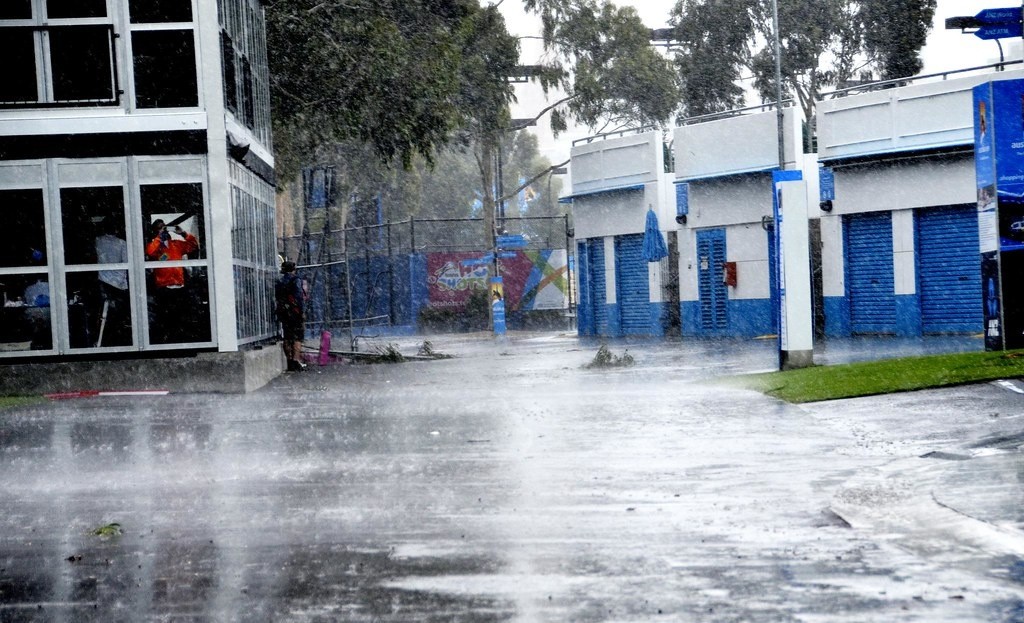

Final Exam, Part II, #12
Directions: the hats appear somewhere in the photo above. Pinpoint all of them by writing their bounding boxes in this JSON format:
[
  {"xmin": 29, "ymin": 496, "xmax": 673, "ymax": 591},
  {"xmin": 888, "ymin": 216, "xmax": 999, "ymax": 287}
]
[{"xmin": 279, "ymin": 262, "xmax": 296, "ymax": 273}]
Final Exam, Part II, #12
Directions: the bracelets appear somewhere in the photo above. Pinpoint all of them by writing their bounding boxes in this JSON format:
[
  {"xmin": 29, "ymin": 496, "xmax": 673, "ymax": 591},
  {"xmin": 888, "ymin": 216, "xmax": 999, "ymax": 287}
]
[{"xmin": 181, "ymin": 231, "xmax": 186, "ymax": 237}]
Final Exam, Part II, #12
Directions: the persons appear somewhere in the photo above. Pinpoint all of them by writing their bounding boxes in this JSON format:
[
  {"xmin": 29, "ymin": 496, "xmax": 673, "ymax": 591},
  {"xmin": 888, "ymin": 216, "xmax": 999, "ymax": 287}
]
[
  {"xmin": 492, "ymin": 289, "xmax": 501, "ymax": 301},
  {"xmin": 96, "ymin": 216, "xmax": 131, "ymax": 347},
  {"xmin": 145, "ymin": 219, "xmax": 203, "ymax": 340},
  {"xmin": 24, "ymin": 272, "xmax": 50, "ymax": 308},
  {"xmin": 276, "ymin": 261, "xmax": 309, "ymax": 371}
]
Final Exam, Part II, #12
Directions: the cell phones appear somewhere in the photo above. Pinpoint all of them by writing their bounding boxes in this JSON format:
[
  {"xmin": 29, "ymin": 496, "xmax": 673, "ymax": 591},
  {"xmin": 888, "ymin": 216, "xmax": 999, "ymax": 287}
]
[{"xmin": 166, "ymin": 226, "xmax": 176, "ymax": 232}]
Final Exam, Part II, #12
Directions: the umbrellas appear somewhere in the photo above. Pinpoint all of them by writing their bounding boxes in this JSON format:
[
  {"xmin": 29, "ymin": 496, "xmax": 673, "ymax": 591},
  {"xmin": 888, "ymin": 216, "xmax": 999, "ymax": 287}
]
[{"xmin": 640, "ymin": 204, "xmax": 670, "ymax": 263}]
